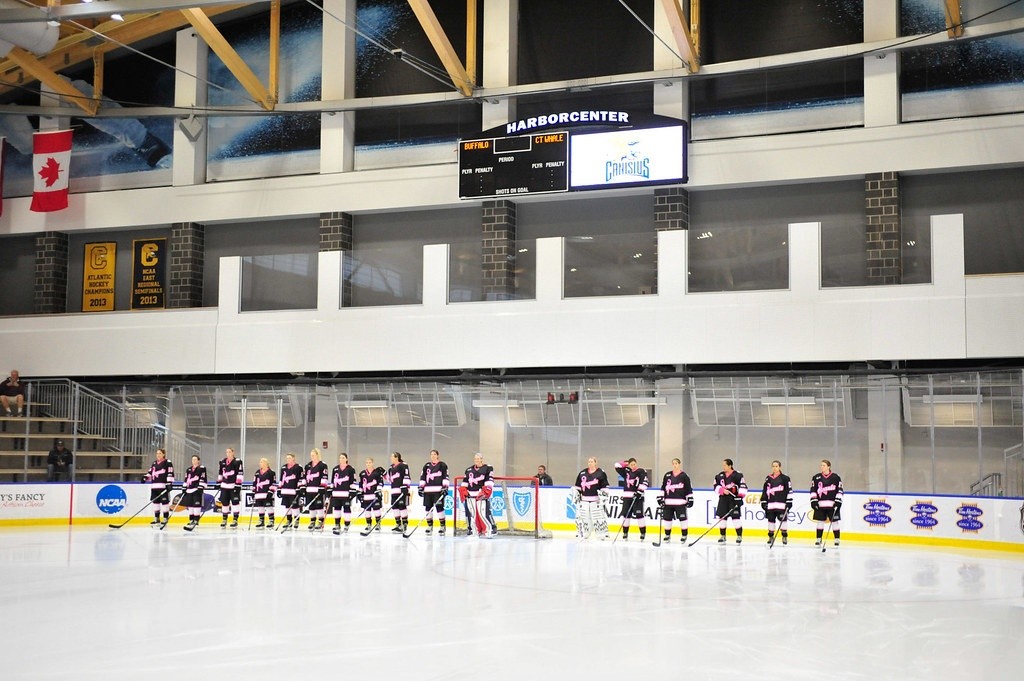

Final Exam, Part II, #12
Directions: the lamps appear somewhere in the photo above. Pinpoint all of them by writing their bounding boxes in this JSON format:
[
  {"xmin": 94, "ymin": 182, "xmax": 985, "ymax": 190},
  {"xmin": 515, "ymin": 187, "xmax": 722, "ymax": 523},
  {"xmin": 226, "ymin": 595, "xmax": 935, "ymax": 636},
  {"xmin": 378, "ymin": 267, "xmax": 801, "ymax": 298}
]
[
  {"xmin": 228, "ymin": 401, "xmax": 270, "ymax": 410},
  {"xmin": 472, "ymin": 400, "xmax": 519, "ymax": 408},
  {"xmin": 345, "ymin": 401, "xmax": 389, "ymax": 408},
  {"xmin": 120, "ymin": 398, "xmax": 159, "ymax": 409},
  {"xmin": 922, "ymin": 394, "xmax": 983, "ymax": 404},
  {"xmin": 617, "ymin": 397, "xmax": 667, "ymax": 405},
  {"xmin": 761, "ymin": 396, "xmax": 817, "ymax": 405}
]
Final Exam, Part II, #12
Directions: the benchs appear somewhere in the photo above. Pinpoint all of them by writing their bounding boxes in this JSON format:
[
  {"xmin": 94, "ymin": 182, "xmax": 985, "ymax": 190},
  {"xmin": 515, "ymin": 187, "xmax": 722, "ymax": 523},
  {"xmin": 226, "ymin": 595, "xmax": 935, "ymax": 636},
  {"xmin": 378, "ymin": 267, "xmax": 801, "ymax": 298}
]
[{"xmin": 0, "ymin": 401, "xmax": 150, "ymax": 478}]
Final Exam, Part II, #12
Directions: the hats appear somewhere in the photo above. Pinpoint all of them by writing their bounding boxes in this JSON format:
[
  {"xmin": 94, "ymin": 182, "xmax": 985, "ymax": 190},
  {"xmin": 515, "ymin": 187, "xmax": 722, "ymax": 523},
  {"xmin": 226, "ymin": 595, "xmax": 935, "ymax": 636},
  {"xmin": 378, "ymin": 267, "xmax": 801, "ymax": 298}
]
[{"xmin": 57, "ymin": 440, "xmax": 63, "ymax": 446}]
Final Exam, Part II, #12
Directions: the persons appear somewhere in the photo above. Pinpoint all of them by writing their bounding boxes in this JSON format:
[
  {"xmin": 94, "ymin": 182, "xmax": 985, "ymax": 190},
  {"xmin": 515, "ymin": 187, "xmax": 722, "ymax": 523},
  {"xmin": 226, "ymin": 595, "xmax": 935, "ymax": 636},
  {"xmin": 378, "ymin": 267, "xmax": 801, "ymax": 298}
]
[
  {"xmin": 217, "ymin": 448, "xmax": 244, "ymax": 529},
  {"xmin": 461, "ymin": 452, "xmax": 498, "ymax": 539},
  {"xmin": 0, "ymin": 68, "xmax": 172, "ymax": 168},
  {"xmin": 713, "ymin": 458, "xmax": 748, "ymax": 546},
  {"xmin": 251, "ymin": 458, "xmax": 276, "ymax": 529},
  {"xmin": 277, "ymin": 448, "xmax": 411, "ymax": 534},
  {"xmin": 141, "ymin": 448, "xmax": 174, "ymax": 525},
  {"xmin": 0, "ymin": 370, "xmax": 24, "ymax": 417},
  {"xmin": 182, "ymin": 455, "xmax": 207, "ymax": 527},
  {"xmin": 614, "ymin": 458, "xmax": 649, "ymax": 542},
  {"xmin": 531, "ymin": 465, "xmax": 553, "ymax": 486},
  {"xmin": 47, "ymin": 440, "xmax": 73, "ymax": 482},
  {"xmin": 656, "ymin": 457, "xmax": 694, "ymax": 544},
  {"xmin": 417, "ymin": 449, "xmax": 450, "ymax": 536},
  {"xmin": 760, "ymin": 460, "xmax": 794, "ymax": 547},
  {"xmin": 810, "ymin": 460, "xmax": 844, "ymax": 549},
  {"xmin": 573, "ymin": 457, "xmax": 610, "ymax": 543}
]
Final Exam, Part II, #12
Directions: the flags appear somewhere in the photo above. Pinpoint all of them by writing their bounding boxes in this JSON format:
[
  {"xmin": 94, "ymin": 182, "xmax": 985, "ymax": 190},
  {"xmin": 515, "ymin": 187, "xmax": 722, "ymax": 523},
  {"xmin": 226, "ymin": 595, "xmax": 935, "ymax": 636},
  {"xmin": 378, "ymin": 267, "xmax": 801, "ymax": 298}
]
[
  {"xmin": 30, "ymin": 130, "xmax": 73, "ymax": 212},
  {"xmin": 0, "ymin": 137, "xmax": 6, "ymax": 217}
]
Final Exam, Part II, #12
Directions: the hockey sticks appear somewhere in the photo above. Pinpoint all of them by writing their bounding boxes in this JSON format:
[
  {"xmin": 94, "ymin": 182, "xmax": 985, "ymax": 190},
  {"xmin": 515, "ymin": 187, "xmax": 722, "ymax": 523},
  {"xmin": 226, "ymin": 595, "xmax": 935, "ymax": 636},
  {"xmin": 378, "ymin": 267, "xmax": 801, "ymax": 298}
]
[
  {"xmin": 688, "ymin": 505, "xmax": 738, "ymax": 547},
  {"xmin": 274, "ymin": 493, "xmax": 320, "ymax": 534},
  {"xmin": 402, "ymin": 494, "xmax": 444, "ymax": 538},
  {"xmin": 769, "ymin": 507, "xmax": 788, "ymax": 549},
  {"xmin": 275, "ymin": 494, "xmax": 298, "ymax": 532},
  {"xmin": 248, "ymin": 497, "xmax": 256, "ymax": 531},
  {"xmin": 187, "ymin": 489, "xmax": 220, "ymax": 525},
  {"xmin": 332, "ymin": 498, "xmax": 376, "ymax": 535},
  {"xmin": 576, "ymin": 501, "xmax": 585, "ymax": 538},
  {"xmin": 360, "ymin": 493, "xmax": 404, "ymax": 537},
  {"xmin": 468, "ymin": 494, "xmax": 485, "ymax": 499},
  {"xmin": 108, "ymin": 489, "xmax": 168, "ymax": 528},
  {"xmin": 652, "ymin": 506, "xmax": 663, "ymax": 547},
  {"xmin": 822, "ymin": 507, "xmax": 838, "ymax": 552},
  {"xmin": 612, "ymin": 497, "xmax": 637, "ymax": 544},
  {"xmin": 152, "ymin": 493, "xmax": 185, "ymax": 530}
]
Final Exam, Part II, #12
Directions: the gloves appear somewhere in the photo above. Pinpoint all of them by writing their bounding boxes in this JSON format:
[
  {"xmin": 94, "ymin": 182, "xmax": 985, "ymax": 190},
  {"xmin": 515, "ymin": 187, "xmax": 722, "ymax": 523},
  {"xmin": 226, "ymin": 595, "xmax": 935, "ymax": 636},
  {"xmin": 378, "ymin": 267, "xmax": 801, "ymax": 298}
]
[
  {"xmin": 327, "ymin": 488, "xmax": 332, "ymax": 497},
  {"xmin": 349, "ymin": 490, "xmax": 357, "ymax": 499},
  {"xmin": 166, "ymin": 482, "xmax": 172, "ymax": 490},
  {"xmin": 401, "ymin": 486, "xmax": 409, "ymax": 496},
  {"xmin": 276, "ymin": 490, "xmax": 281, "ymax": 498},
  {"xmin": 357, "ymin": 492, "xmax": 363, "ymax": 501},
  {"xmin": 295, "ymin": 489, "xmax": 300, "ymax": 497},
  {"xmin": 631, "ymin": 489, "xmax": 841, "ymax": 513},
  {"xmin": 441, "ymin": 487, "xmax": 447, "ymax": 496},
  {"xmin": 198, "ymin": 485, "xmax": 204, "ymax": 494},
  {"xmin": 318, "ymin": 486, "xmax": 325, "ymax": 495},
  {"xmin": 215, "ymin": 483, "xmax": 221, "ymax": 490},
  {"xmin": 375, "ymin": 491, "xmax": 383, "ymax": 500},
  {"xmin": 181, "ymin": 487, "xmax": 187, "ymax": 493},
  {"xmin": 234, "ymin": 486, "xmax": 240, "ymax": 494},
  {"xmin": 300, "ymin": 487, "xmax": 305, "ymax": 496},
  {"xmin": 267, "ymin": 490, "xmax": 273, "ymax": 498},
  {"xmin": 418, "ymin": 487, "xmax": 423, "ymax": 496},
  {"xmin": 141, "ymin": 475, "xmax": 149, "ymax": 483}
]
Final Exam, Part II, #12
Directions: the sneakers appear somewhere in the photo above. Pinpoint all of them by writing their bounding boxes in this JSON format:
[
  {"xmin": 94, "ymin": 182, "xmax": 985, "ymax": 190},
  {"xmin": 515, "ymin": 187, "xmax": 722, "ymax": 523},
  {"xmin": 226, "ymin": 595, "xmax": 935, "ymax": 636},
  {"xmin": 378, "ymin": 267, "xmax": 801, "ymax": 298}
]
[
  {"xmin": 151, "ymin": 519, "xmax": 446, "ymax": 534},
  {"xmin": 623, "ymin": 534, "xmax": 840, "ymax": 548}
]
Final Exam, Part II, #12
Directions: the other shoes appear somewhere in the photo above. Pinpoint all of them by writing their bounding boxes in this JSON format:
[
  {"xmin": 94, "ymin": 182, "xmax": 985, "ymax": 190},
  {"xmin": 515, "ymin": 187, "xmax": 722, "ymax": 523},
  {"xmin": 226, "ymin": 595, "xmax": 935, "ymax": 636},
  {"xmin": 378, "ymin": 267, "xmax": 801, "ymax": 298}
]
[
  {"xmin": 6, "ymin": 412, "xmax": 12, "ymax": 417},
  {"xmin": 17, "ymin": 412, "xmax": 24, "ymax": 417}
]
